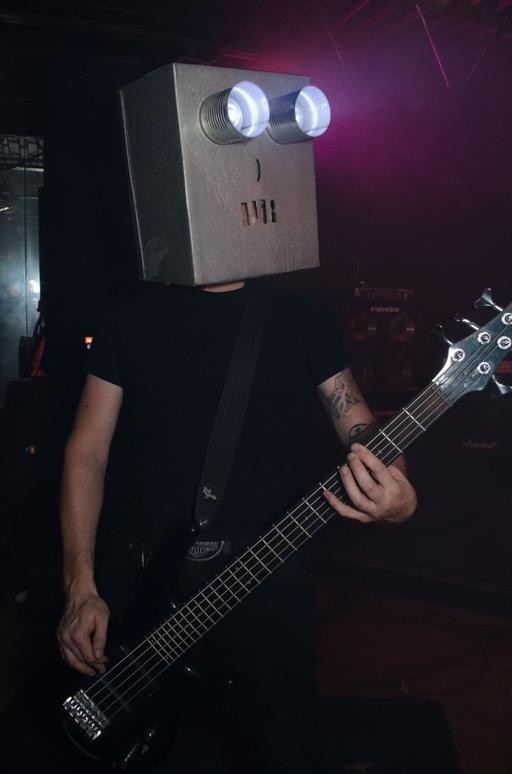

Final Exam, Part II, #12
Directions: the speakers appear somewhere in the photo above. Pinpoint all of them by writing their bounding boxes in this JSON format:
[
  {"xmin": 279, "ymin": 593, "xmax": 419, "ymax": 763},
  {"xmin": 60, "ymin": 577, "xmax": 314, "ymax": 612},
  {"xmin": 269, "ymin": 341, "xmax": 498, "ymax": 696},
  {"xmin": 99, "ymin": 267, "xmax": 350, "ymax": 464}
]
[{"xmin": 347, "ymin": 301, "xmax": 425, "ymax": 402}]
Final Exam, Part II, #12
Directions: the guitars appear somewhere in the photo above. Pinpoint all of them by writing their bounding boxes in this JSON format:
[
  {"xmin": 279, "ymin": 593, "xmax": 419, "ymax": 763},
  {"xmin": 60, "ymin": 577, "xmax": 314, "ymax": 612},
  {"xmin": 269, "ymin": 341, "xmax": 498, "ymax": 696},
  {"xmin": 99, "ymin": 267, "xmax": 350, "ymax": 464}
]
[{"xmin": 2, "ymin": 287, "xmax": 509, "ymax": 771}]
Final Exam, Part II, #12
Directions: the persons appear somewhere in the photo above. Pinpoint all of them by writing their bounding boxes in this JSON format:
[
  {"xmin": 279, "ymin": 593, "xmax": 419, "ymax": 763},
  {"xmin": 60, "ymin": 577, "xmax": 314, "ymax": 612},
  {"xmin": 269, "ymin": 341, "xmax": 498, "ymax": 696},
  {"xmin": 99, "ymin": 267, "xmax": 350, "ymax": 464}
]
[{"xmin": 54, "ymin": 201, "xmax": 421, "ymax": 774}]
